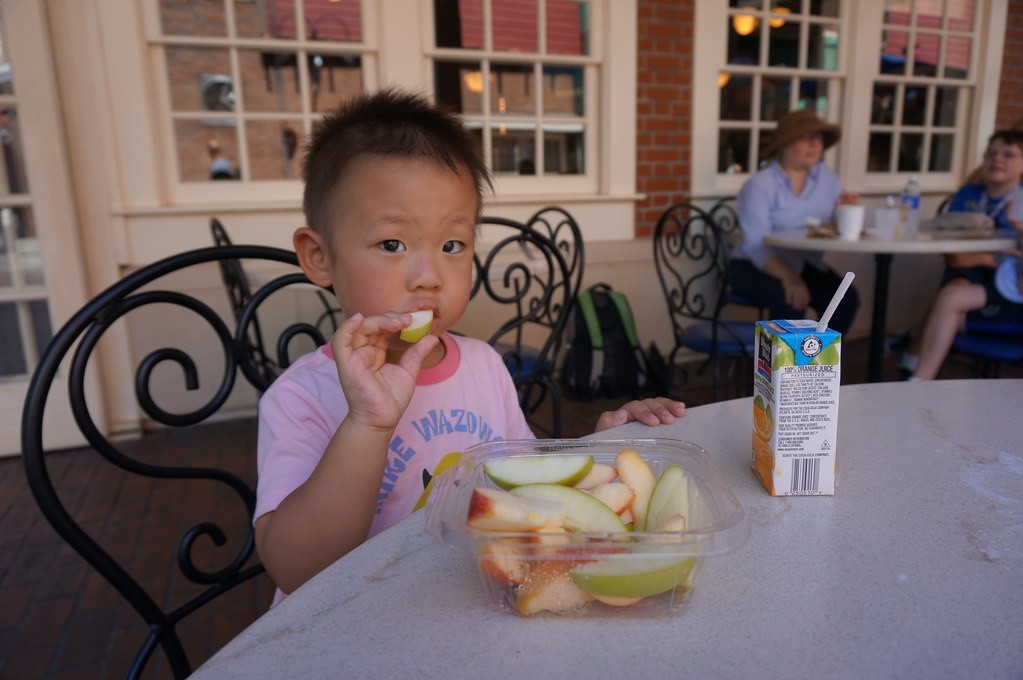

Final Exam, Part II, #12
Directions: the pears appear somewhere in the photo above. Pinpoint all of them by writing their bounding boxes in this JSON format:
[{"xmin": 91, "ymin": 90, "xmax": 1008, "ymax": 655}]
[
  {"xmin": 570, "ymin": 464, "xmax": 695, "ymax": 597},
  {"xmin": 400, "ymin": 308, "xmax": 435, "ymax": 344},
  {"xmin": 480, "ymin": 454, "xmax": 594, "ymax": 490}
]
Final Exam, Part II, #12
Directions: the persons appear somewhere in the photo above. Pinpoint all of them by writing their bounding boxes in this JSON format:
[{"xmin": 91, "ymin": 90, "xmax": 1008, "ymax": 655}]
[
  {"xmin": 206, "ymin": 140, "xmax": 238, "ymax": 180},
  {"xmin": 729, "ymin": 110, "xmax": 862, "ymax": 339},
  {"xmin": 896, "ymin": 128, "xmax": 1023, "ymax": 381},
  {"xmin": 249, "ymin": 87, "xmax": 683, "ymax": 619}
]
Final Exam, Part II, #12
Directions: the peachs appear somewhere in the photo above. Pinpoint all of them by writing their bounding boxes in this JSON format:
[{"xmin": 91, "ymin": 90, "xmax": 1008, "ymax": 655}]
[
  {"xmin": 575, "ymin": 449, "xmax": 657, "ymax": 533},
  {"xmin": 466, "ymin": 487, "xmax": 588, "ymax": 615}
]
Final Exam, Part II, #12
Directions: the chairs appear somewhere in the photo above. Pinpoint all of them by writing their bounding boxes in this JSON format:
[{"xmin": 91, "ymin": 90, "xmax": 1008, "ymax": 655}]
[
  {"xmin": 21, "ymin": 246, "xmax": 344, "ymax": 680},
  {"xmin": 707, "ymin": 196, "xmax": 768, "ymax": 323},
  {"xmin": 448, "ymin": 207, "xmax": 584, "ymax": 438},
  {"xmin": 209, "ymin": 216, "xmax": 267, "ymax": 391},
  {"xmin": 654, "ymin": 204, "xmax": 756, "ymax": 404}
]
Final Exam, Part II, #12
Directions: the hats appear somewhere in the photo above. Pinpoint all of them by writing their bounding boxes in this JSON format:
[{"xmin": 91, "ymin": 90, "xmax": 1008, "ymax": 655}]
[{"xmin": 760, "ymin": 108, "xmax": 843, "ymax": 158}]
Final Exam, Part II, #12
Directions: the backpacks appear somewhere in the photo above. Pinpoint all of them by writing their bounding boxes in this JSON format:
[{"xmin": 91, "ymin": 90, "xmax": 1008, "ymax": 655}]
[{"xmin": 559, "ymin": 280, "xmax": 653, "ymax": 402}]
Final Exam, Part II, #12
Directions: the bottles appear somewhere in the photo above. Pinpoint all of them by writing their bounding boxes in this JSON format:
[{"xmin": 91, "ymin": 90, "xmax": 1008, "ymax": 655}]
[{"xmin": 901, "ymin": 178, "xmax": 920, "ymax": 242}]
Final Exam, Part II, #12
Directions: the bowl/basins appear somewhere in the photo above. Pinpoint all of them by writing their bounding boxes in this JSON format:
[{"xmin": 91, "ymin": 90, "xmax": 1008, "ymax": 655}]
[{"xmin": 435, "ymin": 434, "xmax": 750, "ymax": 621}]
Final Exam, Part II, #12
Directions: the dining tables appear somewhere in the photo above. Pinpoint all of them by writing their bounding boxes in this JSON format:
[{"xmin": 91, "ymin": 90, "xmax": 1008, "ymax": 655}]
[
  {"xmin": 179, "ymin": 380, "xmax": 1023, "ymax": 680},
  {"xmin": 763, "ymin": 221, "xmax": 1018, "ymax": 383}
]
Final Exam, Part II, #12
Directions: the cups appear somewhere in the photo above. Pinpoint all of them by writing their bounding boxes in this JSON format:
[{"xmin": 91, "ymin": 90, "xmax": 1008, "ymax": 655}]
[
  {"xmin": 877, "ymin": 208, "xmax": 900, "ymax": 241},
  {"xmin": 836, "ymin": 205, "xmax": 867, "ymax": 242}
]
